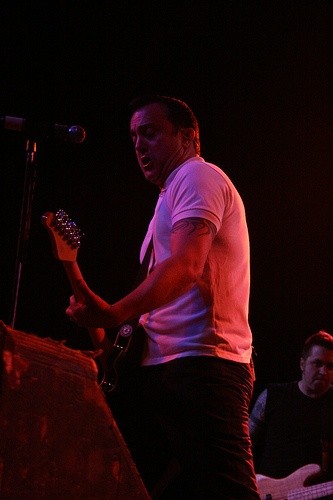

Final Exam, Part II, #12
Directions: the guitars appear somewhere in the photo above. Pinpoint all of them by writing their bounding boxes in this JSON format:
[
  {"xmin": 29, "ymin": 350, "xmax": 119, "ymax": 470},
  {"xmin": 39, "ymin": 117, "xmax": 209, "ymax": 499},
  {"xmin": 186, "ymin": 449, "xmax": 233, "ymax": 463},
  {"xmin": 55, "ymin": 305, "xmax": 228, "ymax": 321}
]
[
  {"xmin": 255, "ymin": 464, "xmax": 333, "ymax": 500},
  {"xmin": 39, "ymin": 209, "xmax": 133, "ymax": 392}
]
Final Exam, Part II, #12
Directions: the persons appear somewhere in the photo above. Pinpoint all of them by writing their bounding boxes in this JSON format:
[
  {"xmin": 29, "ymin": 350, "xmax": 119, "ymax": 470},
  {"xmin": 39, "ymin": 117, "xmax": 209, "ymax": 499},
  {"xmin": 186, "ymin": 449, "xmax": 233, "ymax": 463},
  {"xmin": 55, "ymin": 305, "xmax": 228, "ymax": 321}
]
[
  {"xmin": 66, "ymin": 97, "xmax": 261, "ymax": 500},
  {"xmin": 248, "ymin": 331, "xmax": 333, "ymax": 500}
]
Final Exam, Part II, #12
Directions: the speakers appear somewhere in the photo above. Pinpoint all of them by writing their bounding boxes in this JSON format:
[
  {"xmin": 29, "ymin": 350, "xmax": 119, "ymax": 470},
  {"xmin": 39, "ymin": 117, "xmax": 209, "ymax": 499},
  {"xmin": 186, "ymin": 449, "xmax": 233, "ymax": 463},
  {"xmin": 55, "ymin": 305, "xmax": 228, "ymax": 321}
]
[{"xmin": 0, "ymin": 318, "xmax": 153, "ymax": 500}]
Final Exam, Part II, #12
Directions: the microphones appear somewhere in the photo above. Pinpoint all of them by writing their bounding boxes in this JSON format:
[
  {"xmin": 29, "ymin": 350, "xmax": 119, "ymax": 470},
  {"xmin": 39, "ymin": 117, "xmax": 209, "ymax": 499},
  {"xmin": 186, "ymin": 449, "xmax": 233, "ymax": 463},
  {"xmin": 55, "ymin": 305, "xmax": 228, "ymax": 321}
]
[{"xmin": 0, "ymin": 114, "xmax": 86, "ymax": 144}]
